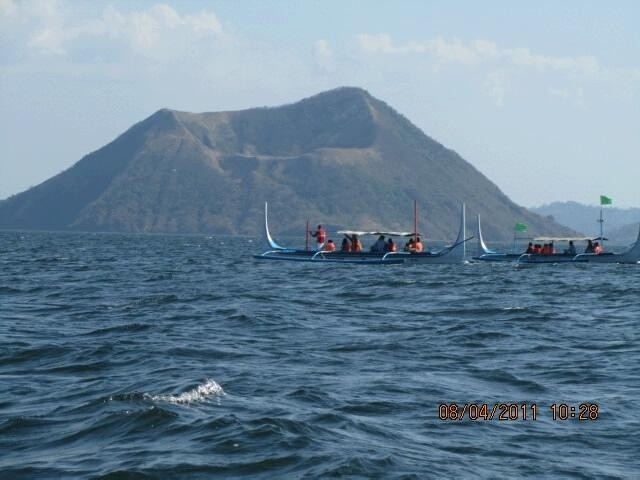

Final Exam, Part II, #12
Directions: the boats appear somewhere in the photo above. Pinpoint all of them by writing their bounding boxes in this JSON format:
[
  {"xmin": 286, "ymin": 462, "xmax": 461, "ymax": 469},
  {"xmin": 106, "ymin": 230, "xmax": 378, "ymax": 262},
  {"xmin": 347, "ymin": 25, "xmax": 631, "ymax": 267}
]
[
  {"xmin": 252, "ymin": 196, "xmax": 466, "ymax": 264},
  {"xmin": 472, "ymin": 213, "xmax": 640, "ymax": 265}
]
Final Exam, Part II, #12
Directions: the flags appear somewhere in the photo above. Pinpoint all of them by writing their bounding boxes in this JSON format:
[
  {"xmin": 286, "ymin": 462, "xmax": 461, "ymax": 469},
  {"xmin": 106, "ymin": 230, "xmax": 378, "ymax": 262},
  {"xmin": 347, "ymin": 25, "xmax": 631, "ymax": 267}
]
[
  {"xmin": 601, "ymin": 195, "xmax": 613, "ymax": 205},
  {"xmin": 515, "ymin": 223, "xmax": 528, "ymax": 232}
]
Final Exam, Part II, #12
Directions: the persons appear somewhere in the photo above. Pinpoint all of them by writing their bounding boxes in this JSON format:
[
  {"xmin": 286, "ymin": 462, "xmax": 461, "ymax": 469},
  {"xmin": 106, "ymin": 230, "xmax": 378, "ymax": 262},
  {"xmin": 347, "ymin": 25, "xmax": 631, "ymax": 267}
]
[
  {"xmin": 406, "ymin": 237, "xmax": 424, "ymax": 253},
  {"xmin": 372, "ymin": 236, "xmax": 388, "ymax": 252},
  {"xmin": 309, "ymin": 224, "xmax": 326, "ymax": 251},
  {"xmin": 585, "ymin": 240, "xmax": 602, "ymax": 254},
  {"xmin": 526, "ymin": 242, "xmax": 555, "ymax": 255},
  {"xmin": 388, "ymin": 238, "xmax": 397, "ymax": 252},
  {"xmin": 342, "ymin": 234, "xmax": 363, "ymax": 253},
  {"xmin": 324, "ymin": 239, "xmax": 335, "ymax": 251},
  {"xmin": 565, "ymin": 241, "xmax": 576, "ymax": 256}
]
